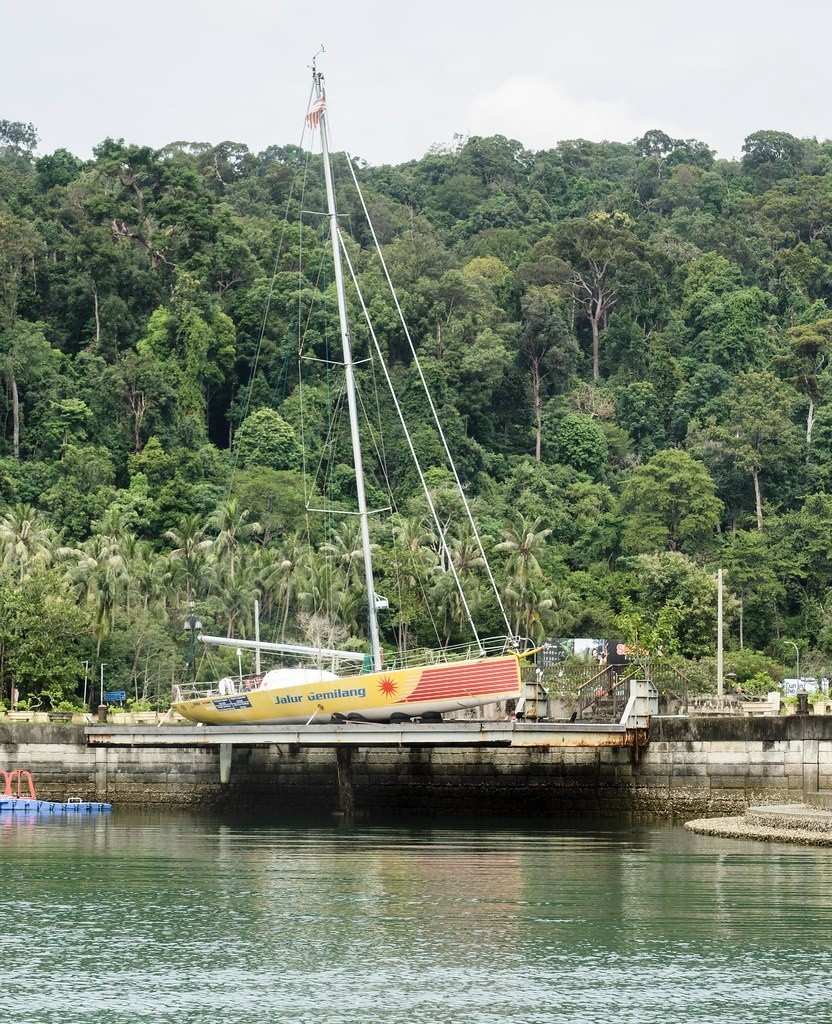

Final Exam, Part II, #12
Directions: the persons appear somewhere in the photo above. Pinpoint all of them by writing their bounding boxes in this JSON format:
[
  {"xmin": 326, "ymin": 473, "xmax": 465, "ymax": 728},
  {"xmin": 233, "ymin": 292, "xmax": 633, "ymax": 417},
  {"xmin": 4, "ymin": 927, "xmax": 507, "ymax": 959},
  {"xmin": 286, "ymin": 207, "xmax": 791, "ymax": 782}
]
[
  {"xmin": 526, "ymin": 701, "xmax": 537, "ymax": 723},
  {"xmin": 590, "ymin": 648, "xmax": 603, "ymax": 669},
  {"xmin": 509, "ymin": 707, "xmax": 517, "ymax": 723}
]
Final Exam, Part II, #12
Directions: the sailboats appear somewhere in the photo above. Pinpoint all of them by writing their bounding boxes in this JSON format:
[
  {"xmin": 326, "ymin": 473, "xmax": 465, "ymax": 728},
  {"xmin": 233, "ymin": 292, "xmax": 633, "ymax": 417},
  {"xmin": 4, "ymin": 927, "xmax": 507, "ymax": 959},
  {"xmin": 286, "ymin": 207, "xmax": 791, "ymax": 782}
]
[{"xmin": 165, "ymin": 45, "xmax": 544, "ymax": 729}]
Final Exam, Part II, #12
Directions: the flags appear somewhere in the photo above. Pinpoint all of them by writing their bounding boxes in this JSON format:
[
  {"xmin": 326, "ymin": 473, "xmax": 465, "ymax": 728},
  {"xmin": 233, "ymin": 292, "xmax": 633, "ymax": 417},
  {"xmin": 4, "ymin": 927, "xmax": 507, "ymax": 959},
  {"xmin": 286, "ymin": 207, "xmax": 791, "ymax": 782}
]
[{"xmin": 306, "ymin": 104, "xmax": 325, "ymax": 126}]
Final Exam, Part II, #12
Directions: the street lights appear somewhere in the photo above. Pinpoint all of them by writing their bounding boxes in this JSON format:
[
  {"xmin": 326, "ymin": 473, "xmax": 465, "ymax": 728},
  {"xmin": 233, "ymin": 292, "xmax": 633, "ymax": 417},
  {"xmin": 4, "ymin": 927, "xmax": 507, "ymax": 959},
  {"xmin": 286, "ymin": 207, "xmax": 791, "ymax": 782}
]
[
  {"xmin": 182, "ymin": 599, "xmax": 202, "ymax": 683},
  {"xmin": 81, "ymin": 660, "xmax": 89, "ymax": 712},
  {"xmin": 97, "ymin": 663, "xmax": 111, "ymax": 723},
  {"xmin": 783, "ymin": 641, "xmax": 798, "ymax": 692}
]
